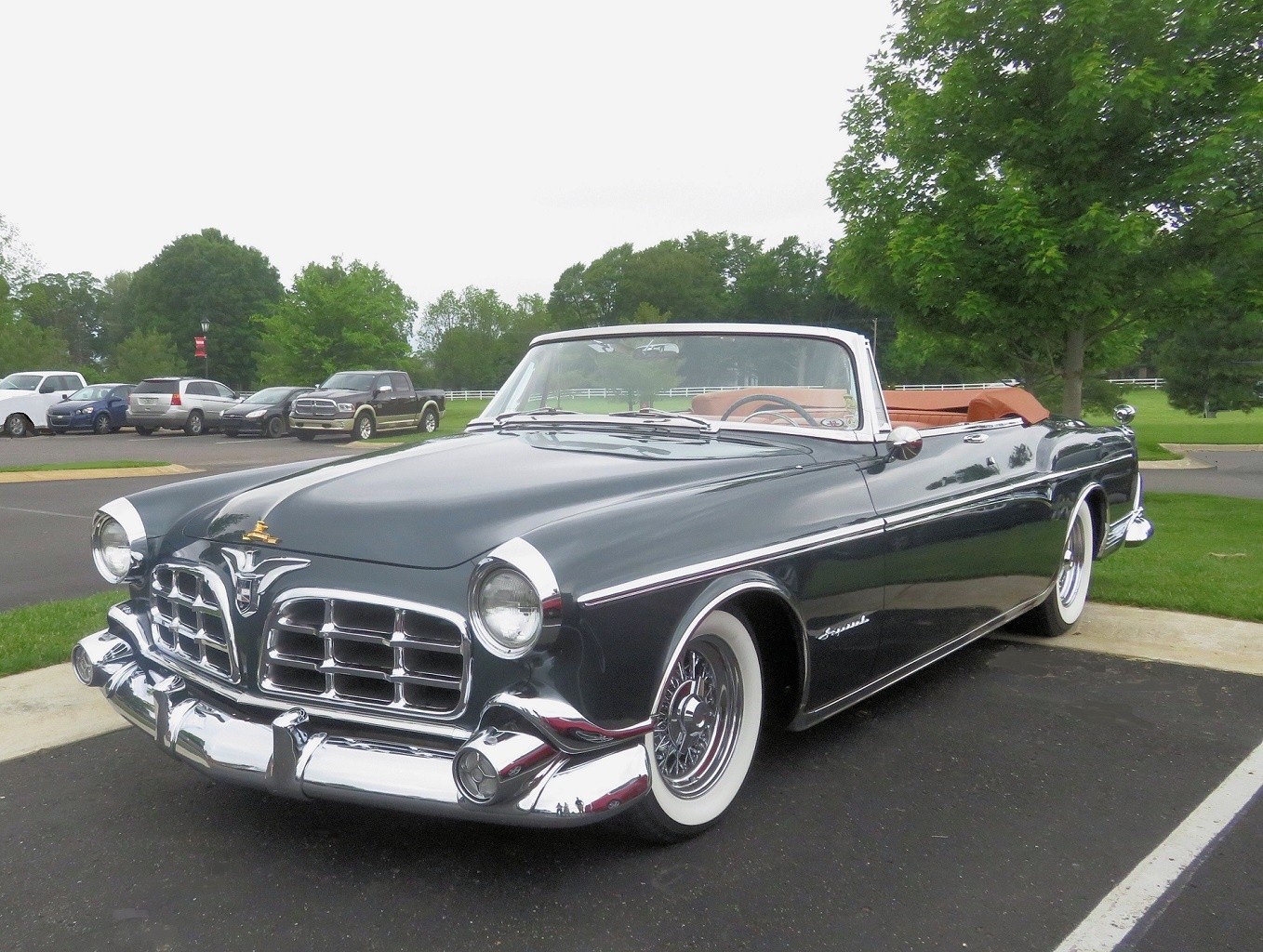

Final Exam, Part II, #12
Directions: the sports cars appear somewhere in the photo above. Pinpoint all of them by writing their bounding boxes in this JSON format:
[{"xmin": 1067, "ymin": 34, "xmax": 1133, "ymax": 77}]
[{"xmin": 70, "ymin": 320, "xmax": 1154, "ymax": 844}]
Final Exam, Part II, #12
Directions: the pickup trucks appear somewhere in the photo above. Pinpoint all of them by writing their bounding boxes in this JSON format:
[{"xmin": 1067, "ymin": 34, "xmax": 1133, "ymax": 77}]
[{"xmin": 288, "ymin": 369, "xmax": 447, "ymax": 441}]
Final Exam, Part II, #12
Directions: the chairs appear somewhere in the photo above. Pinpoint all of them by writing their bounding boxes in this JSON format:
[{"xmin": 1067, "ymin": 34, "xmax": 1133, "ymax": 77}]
[{"xmin": 759, "ymin": 409, "xmax": 965, "ymax": 428}]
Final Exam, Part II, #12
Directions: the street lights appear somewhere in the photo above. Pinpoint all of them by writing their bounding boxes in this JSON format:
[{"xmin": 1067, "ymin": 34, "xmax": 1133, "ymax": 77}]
[{"xmin": 199, "ymin": 317, "xmax": 212, "ymax": 379}]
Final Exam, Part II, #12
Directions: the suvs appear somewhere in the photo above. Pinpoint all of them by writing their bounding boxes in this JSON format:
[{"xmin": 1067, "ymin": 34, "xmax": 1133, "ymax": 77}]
[
  {"xmin": 0, "ymin": 372, "xmax": 88, "ymax": 439},
  {"xmin": 126, "ymin": 376, "xmax": 247, "ymax": 436}
]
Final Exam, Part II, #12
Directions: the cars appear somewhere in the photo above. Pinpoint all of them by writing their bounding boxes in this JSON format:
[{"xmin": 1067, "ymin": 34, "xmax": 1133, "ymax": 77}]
[
  {"xmin": 45, "ymin": 384, "xmax": 138, "ymax": 435},
  {"xmin": 221, "ymin": 386, "xmax": 319, "ymax": 438}
]
